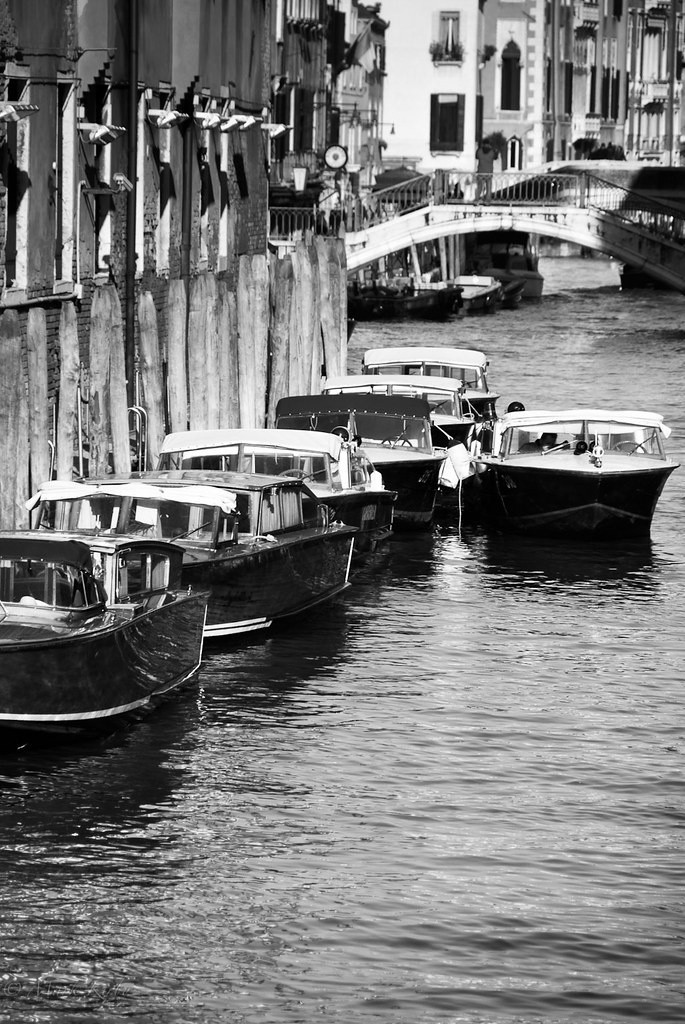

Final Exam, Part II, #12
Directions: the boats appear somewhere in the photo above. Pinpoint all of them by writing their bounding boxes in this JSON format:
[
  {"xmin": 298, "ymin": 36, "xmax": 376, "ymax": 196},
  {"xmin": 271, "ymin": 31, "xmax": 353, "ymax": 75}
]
[
  {"xmin": 322, "ymin": 346, "xmax": 500, "ymax": 451},
  {"xmin": 471, "ymin": 410, "xmax": 681, "ymax": 541},
  {"xmin": 1, "ymin": 527, "xmax": 211, "ymax": 722},
  {"xmin": 152, "ymin": 428, "xmax": 399, "ymax": 562},
  {"xmin": 479, "ymin": 244, "xmax": 545, "ymax": 297},
  {"xmin": 35, "ymin": 470, "xmax": 360, "ymax": 637},
  {"xmin": 274, "ymin": 394, "xmax": 450, "ymax": 523},
  {"xmin": 346, "ymin": 275, "xmax": 527, "ymax": 343}
]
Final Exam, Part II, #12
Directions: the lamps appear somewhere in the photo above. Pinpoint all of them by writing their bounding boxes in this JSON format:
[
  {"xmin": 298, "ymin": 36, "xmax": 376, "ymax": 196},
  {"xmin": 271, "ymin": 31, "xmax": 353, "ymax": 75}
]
[
  {"xmin": 77, "ymin": 122, "xmax": 126, "ymax": 145},
  {"xmin": 148, "ymin": 109, "xmax": 189, "ymax": 129},
  {"xmin": 0, "ymin": 103, "xmax": 41, "ymax": 122},
  {"xmin": 195, "ymin": 111, "xmax": 289, "ymax": 138}
]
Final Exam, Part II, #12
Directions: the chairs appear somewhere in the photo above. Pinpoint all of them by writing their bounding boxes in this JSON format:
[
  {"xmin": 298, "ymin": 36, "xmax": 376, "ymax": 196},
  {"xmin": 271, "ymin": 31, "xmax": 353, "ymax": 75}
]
[
  {"xmin": 428, "ymin": 402, "xmax": 447, "ymax": 415},
  {"xmin": 376, "ymin": 420, "xmax": 410, "ymax": 440},
  {"xmin": 11, "ymin": 576, "xmax": 72, "ymax": 607}
]
